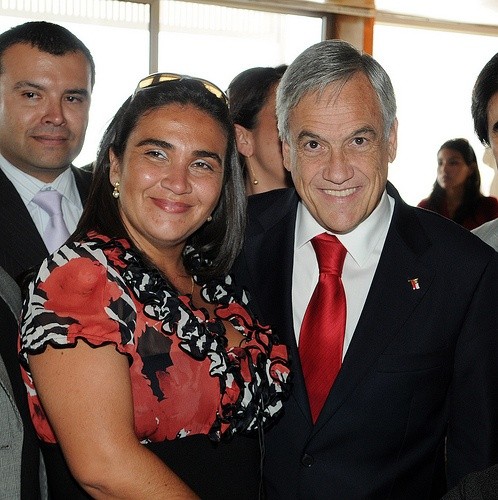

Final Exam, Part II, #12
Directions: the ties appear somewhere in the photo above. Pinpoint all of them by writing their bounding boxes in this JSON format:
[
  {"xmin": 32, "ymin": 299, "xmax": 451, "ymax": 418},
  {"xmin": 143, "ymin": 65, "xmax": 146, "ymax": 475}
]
[
  {"xmin": 30, "ymin": 190, "xmax": 71, "ymax": 256},
  {"xmin": 296, "ymin": 232, "xmax": 347, "ymax": 425}
]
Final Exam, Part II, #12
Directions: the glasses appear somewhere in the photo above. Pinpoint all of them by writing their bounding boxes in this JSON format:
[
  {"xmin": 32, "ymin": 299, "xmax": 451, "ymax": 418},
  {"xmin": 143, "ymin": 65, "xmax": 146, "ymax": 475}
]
[{"xmin": 109, "ymin": 72, "xmax": 230, "ymax": 144}]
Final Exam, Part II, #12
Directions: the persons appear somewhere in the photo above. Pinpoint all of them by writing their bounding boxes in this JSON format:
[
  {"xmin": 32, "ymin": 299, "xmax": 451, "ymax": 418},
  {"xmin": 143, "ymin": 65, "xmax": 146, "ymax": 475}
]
[
  {"xmin": 16, "ymin": 73, "xmax": 290, "ymax": 500},
  {"xmin": 470, "ymin": 53, "xmax": 498, "ymax": 253},
  {"xmin": 227, "ymin": 65, "xmax": 289, "ymax": 205},
  {"xmin": 416, "ymin": 138, "xmax": 498, "ymax": 231},
  {"xmin": 0, "ymin": 21, "xmax": 95, "ymax": 500},
  {"xmin": 230, "ymin": 40, "xmax": 498, "ymax": 500}
]
